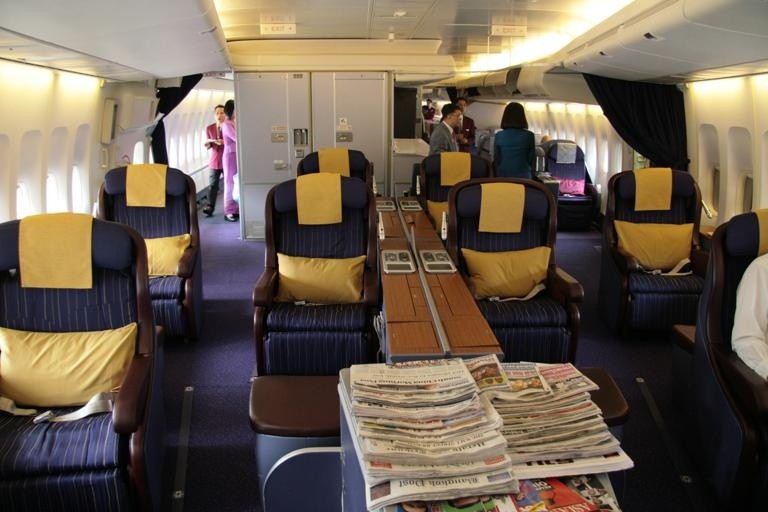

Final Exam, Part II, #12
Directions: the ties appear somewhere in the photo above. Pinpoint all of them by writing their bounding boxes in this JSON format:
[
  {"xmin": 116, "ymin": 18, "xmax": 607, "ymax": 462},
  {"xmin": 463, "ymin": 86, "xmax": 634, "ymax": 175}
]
[{"xmin": 217, "ymin": 126, "xmax": 222, "ymax": 140}]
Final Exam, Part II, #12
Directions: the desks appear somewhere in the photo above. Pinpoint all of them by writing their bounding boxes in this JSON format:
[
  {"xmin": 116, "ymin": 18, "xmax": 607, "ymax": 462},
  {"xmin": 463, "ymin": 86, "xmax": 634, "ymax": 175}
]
[
  {"xmin": 571, "ymin": 363, "xmax": 631, "ymax": 454},
  {"xmin": 248, "ymin": 373, "xmax": 373, "ymax": 511}
]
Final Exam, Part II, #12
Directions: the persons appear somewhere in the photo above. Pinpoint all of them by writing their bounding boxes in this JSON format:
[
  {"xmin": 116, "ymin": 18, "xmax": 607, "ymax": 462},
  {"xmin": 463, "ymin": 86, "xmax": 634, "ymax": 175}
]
[
  {"xmin": 453, "ymin": 98, "xmax": 475, "ymax": 151},
  {"xmin": 493, "ymin": 103, "xmax": 536, "ymax": 179},
  {"xmin": 422, "ymin": 100, "xmax": 435, "ymax": 120},
  {"xmin": 728, "ymin": 254, "xmax": 766, "ymax": 511},
  {"xmin": 221, "ymin": 100, "xmax": 240, "ymax": 222},
  {"xmin": 429, "ymin": 103, "xmax": 462, "ymax": 153},
  {"xmin": 203, "ymin": 105, "xmax": 225, "ymax": 215}
]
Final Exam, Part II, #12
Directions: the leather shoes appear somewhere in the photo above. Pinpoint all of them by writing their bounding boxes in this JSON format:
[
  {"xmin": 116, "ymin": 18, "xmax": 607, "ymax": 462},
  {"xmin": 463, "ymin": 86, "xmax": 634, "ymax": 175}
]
[
  {"xmin": 224, "ymin": 215, "xmax": 237, "ymax": 222},
  {"xmin": 202, "ymin": 206, "xmax": 214, "ymax": 217}
]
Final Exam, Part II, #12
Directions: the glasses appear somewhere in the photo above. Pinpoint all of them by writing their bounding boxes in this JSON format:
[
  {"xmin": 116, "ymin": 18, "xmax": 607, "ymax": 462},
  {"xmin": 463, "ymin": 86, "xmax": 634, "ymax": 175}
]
[
  {"xmin": 457, "ymin": 104, "xmax": 466, "ymax": 107},
  {"xmin": 450, "ymin": 114, "xmax": 464, "ymax": 119}
]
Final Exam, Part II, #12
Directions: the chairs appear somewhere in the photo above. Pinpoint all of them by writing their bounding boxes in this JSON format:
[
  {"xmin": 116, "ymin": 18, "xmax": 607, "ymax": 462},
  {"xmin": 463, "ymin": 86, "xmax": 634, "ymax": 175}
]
[
  {"xmin": 97, "ymin": 163, "xmax": 204, "ymax": 348},
  {"xmin": 442, "ymin": 176, "xmax": 583, "ymax": 366},
  {"xmin": 601, "ymin": 168, "xmax": 718, "ymax": 335},
  {"xmin": 412, "ymin": 150, "xmax": 492, "ymax": 240},
  {"xmin": 689, "ymin": 197, "xmax": 768, "ymax": 503},
  {"xmin": 251, "ymin": 171, "xmax": 382, "ymax": 377},
  {"xmin": 542, "ymin": 139, "xmax": 602, "ymax": 230},
  {"xmin": 0, "ymin": 212, "xmax": 168, "ymax": 512},
  {"xmin": 294, "ymin": 148, "xmax": 376, "ymax": 187}
]
